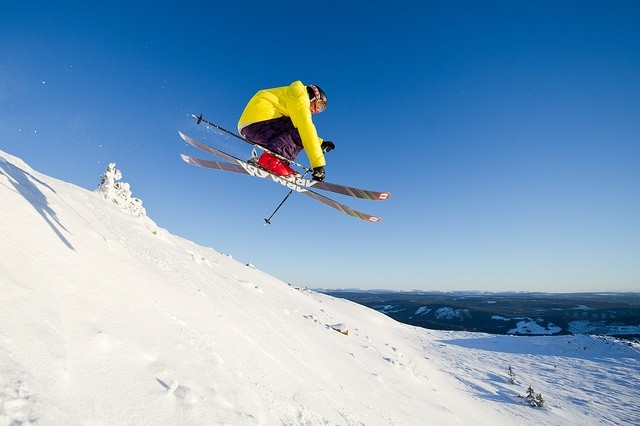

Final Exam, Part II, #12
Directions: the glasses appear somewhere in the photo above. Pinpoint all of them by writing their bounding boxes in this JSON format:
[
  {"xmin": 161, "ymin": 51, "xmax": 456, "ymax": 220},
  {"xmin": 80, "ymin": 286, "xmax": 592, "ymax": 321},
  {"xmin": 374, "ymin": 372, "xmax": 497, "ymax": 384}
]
[{"xmin": 309, "ymin": 84, "xmax": 328, "ymax": 114}]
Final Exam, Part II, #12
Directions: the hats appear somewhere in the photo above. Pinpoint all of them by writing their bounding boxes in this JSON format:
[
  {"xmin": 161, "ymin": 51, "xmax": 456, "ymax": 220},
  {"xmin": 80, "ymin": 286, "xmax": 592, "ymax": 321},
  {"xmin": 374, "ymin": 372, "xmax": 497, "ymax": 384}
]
[{"xmin": 307, "ymin": 83, "xmax": 328, "ymax": 105}]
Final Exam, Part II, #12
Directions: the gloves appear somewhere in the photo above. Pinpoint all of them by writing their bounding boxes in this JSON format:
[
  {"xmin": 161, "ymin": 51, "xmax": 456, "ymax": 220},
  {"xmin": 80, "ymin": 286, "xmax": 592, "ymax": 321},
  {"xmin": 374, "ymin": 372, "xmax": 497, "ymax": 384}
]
[
  {"xmin": 321, "ymin": 140, "xmax": 336, "ymax": 153},
  {"xmin": 312, "ymin": 165, "xmax": 327, "ymax": 183}
]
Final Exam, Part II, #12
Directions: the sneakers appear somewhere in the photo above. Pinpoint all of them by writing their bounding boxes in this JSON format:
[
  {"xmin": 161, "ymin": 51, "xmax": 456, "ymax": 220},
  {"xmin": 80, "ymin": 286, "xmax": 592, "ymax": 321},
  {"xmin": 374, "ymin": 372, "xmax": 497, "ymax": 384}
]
[
  {"xmin": 258, "ymin": 151, "xmax": 290, "ymax": 177},
  {"xmin": 285, "ymin": 166, "xmax": 297, "ymax": 174}
]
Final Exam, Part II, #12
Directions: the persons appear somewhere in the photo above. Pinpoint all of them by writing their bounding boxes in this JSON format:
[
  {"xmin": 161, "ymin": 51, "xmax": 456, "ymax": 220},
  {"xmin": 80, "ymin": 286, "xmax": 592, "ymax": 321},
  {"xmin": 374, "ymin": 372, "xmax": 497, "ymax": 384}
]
[{"xmin": 236, "ymin": 80, "xmax": 335, "ymax": 182}]
